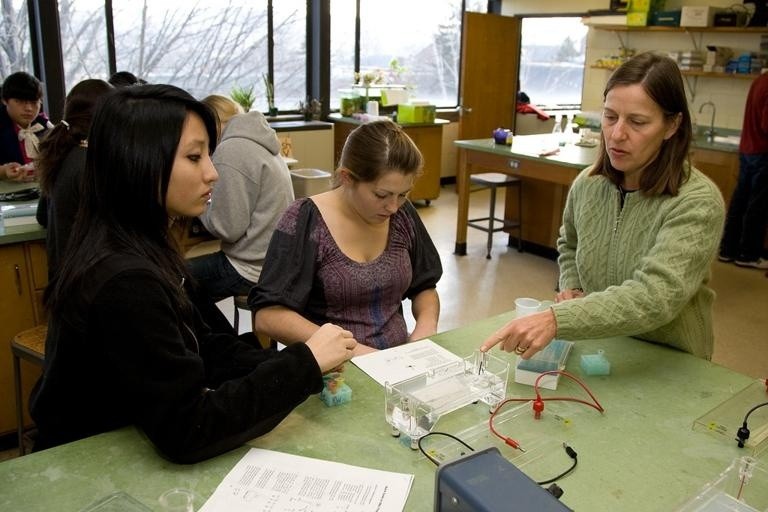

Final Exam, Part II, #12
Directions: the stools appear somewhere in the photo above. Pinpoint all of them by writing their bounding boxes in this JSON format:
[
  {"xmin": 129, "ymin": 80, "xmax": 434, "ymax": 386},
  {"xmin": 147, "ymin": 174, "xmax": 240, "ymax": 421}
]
[
  {"xmin": 9, "ymin": 321, "xmax": 52, "ymax": 456},
  {"xmin": 467, "ymin": 171, "xmax": 522, "ymax": 259}
]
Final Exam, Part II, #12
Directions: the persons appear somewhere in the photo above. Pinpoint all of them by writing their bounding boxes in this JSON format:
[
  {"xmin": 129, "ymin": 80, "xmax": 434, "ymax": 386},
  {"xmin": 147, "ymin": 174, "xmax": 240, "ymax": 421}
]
[
  {"xmin": 27, "ymin": 83, "xmax": 357, "ymax": 465},
  {"xmin": 1, "ymin": 70, "xmax": 49, "ymax": 181},
  {"xmin": 718, "ymin": 70, "xmax": 767, "ymax": 271},
  {"xmin": 477, "ymin": 51, "xmax": 723, "ymax": 358},
  {"xmin": 189, "ymin": 94, "xmax": 295, "ymax": 301},
  {"xmin": 33, "ymin": 71, "xmax": 149, "ymax": 284},
  {"xmin": 253, "ymin": 120, "xmax": 442, "ymax": 351}
]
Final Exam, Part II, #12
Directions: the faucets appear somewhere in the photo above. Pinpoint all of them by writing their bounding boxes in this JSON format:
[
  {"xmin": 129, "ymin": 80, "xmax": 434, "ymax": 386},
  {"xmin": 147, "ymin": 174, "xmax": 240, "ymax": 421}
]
[{"xmin": 699, "ymin": 101, "xmax": 717, "ymax": 139}]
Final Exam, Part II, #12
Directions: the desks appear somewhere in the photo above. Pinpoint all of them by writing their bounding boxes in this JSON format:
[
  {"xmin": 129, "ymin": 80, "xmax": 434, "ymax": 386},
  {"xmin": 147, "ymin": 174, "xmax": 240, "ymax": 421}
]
[
  {"xmin": 0, "ymin": 179, "xmax": 53, "ymax": 437},
  {"xmin": 267, "ymin": 120, "xmax": 335, "ymax": 182},
  {"xmin": 327, "ymin": 110, "xmax": 451, "ymax": 208},
  {"xmin": 0, "ymin": 298, "xmax": 768, "ymax": 512},
  {"xmin": 451, "ymin": 130, "xmax": 604, "ymax": 263}
]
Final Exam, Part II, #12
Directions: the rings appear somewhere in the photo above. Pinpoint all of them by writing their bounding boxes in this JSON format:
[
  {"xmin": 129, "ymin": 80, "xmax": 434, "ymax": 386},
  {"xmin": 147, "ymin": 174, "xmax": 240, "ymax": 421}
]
[{"xmin": 515, "ymin": 345, "xmax": 526, "ymax": 355}]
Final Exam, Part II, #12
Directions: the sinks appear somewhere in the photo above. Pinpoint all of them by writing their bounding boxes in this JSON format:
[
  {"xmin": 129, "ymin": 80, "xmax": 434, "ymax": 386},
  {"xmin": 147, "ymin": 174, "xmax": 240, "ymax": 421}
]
[{"xmin": 706, "ymin": 134, "xmax": 742, "ymax": 146}]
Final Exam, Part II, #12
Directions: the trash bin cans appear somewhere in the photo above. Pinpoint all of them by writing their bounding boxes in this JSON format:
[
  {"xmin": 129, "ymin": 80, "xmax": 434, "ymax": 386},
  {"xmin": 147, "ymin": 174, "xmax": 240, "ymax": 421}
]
[{"xmin": 290, "ymin": 169, "xmax": 332, "ymax": 199}]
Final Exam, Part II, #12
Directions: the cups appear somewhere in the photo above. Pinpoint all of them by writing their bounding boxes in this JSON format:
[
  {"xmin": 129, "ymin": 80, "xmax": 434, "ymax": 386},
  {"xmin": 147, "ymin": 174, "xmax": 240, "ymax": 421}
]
[
  {"xmin": 368, "ymin": 101, "xmax": 379, "ymax": 117},
  {"xmin": 159, "ymin": 488, "xmax": 194, "ymax": 512},
  {"xmin": 514, "ymin": 297, "xmax": 541, "ymax": 317},
  {"xmin": 340, "ymin": 98, "xmax": 361, "ymax": 118},
  {"xmin": 493, "ymin": 131, "xmax": 508, "ymax": 145}
]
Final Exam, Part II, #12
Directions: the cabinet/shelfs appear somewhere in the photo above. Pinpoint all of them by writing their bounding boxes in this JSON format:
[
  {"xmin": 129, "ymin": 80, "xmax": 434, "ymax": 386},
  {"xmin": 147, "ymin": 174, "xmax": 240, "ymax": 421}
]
[{"xmin": 587, "ymin": 24, "xmax": 766, "ymax": 104}]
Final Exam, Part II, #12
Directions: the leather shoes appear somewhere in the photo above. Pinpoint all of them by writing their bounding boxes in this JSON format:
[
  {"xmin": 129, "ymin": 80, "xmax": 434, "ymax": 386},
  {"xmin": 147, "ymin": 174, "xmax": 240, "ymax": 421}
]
[{"xmin": 735, "ymin": 257, "xmax": 768, "ymax": 269}]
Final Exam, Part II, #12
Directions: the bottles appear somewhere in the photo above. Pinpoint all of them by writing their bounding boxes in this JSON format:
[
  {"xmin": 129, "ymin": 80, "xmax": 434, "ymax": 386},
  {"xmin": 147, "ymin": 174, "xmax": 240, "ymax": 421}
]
[{"xmin": 506, "ymin": 132, "xmax": 513, "ymax": 145}]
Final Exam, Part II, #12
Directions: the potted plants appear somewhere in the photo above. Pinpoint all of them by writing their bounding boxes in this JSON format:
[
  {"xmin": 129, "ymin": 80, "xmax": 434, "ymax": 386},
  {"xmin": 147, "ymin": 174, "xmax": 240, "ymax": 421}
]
[
  {"xmin": 232, "ymin": 82, "xmax": 262, "ymax": 114},
  {"xmin": 262, "ymin": 72, "xmax": 280, "ymax": 117}
]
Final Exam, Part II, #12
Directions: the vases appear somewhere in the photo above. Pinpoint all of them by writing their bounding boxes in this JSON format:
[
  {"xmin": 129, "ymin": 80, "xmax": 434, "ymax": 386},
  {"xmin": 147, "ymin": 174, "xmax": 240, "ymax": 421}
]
[{"xmin": 303, "ymin": 112, "xmax": 312, "ymax": 123}]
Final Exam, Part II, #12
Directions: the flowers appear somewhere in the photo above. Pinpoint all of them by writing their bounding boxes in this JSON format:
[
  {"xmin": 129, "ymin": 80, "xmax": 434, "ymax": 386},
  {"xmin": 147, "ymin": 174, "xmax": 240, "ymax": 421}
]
[{"xmin": 296, "ymin": 94, "xmax": 323, "ymax": 114}]
